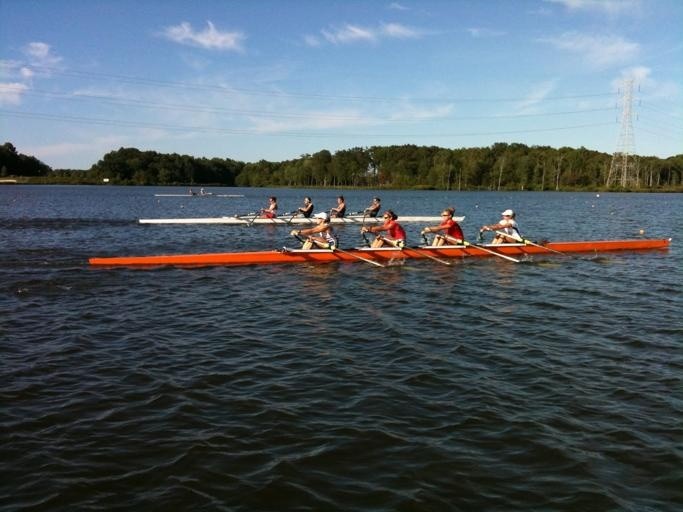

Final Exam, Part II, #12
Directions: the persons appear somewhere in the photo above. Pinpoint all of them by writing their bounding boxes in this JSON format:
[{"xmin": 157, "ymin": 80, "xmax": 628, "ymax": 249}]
[
  {"xmin": 330, "ymin": 196, "xmax": 346, "ymax": 217},
  {"xmin": 361, "ymin": 209, "xmax": 406, "ymax": 249},
  {"xmin": 362, "ymin": 197, "xmax": 381, "ymax": 217},
  {"xmin": 479, "ymin": 208, "xmax": 524, "ymax": 244},
  {"xmin": 289, "ymin": 212, "xmax": 338, "ymax": 250},
  {"xmin": 291, "ymin": 196, "xmax": 314, "ymax": 217},
  {"xmin": 420, "ymin": 207, "xmax": 463, "ymax": 248},
  {"xmin": 256, "ymin": 195, "xmax": 277, "ymax": 219}
]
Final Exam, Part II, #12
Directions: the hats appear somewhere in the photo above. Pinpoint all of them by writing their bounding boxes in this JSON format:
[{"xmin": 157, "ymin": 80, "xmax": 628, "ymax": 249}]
[
  {"xmin": 314, "ymin": 212, "xmax": 331, "ymax": 220},
  {"xmin": 501, "ymin": 208, "xmax": 514, "ymax": 217}
]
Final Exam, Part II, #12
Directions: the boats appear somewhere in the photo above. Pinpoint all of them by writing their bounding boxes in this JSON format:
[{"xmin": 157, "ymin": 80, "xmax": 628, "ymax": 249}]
[
  {"xmin": 135, "ymin": 215, "xmax": 463, "ymax": 229},
  {"xmin": 155, "ymin": 192, "xmax": 244, "ymax": 198},
  {"xmin": 85, "ymin": 237, "xmax": 675, "ymax": 270}
]
